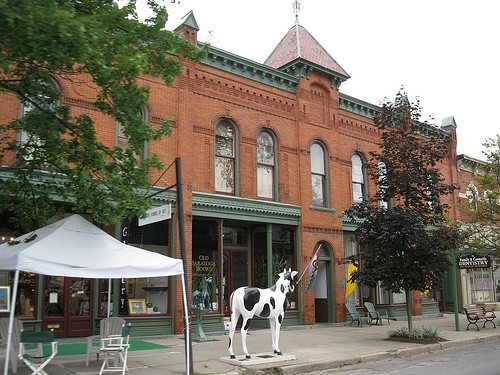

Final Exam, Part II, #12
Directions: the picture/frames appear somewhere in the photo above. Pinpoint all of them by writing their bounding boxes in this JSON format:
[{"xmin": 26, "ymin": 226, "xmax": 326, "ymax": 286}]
[
  {"xmin": 0, "ymin": 286, "xmax": 10, "ymax": 312},
  {"xmin": 129, "ymin": 298, "xmax": 147, "ymax": 313}
]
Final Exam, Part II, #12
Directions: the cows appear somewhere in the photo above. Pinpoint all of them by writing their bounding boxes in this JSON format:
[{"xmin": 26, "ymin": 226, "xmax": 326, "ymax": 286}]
[{"xmin": 227, "ymin": 268, "xmax": 298, "ymax": 359}]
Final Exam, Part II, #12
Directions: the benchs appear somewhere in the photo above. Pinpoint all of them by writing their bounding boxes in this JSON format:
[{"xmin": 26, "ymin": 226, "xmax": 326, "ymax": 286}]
[{"xmin": 461, "ymin": 306, "xmax": 496, "ymax": 331}]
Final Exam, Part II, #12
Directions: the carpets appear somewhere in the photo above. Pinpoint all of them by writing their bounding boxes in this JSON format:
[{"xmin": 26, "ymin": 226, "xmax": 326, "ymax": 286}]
[
  {"xmin": 24, "ymin": 339, "xmax": 173, "ymax": 356},
  {"xmin": 0, "ymin": 363, "xmax": 75, "ymax": 375}
]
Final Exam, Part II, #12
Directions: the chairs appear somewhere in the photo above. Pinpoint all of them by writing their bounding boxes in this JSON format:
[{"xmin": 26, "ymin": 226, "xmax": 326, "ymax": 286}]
[
  {"xmin": 0, "ymin": 318, "xmax": 41, "ymax": 373},
  {"xmin": 86, "ymin": 317, "xmax": 131, "ymax": 375},
  {"xmin": 345, "ymin": 303, "xmax": 390, "ymax": 328}
]
